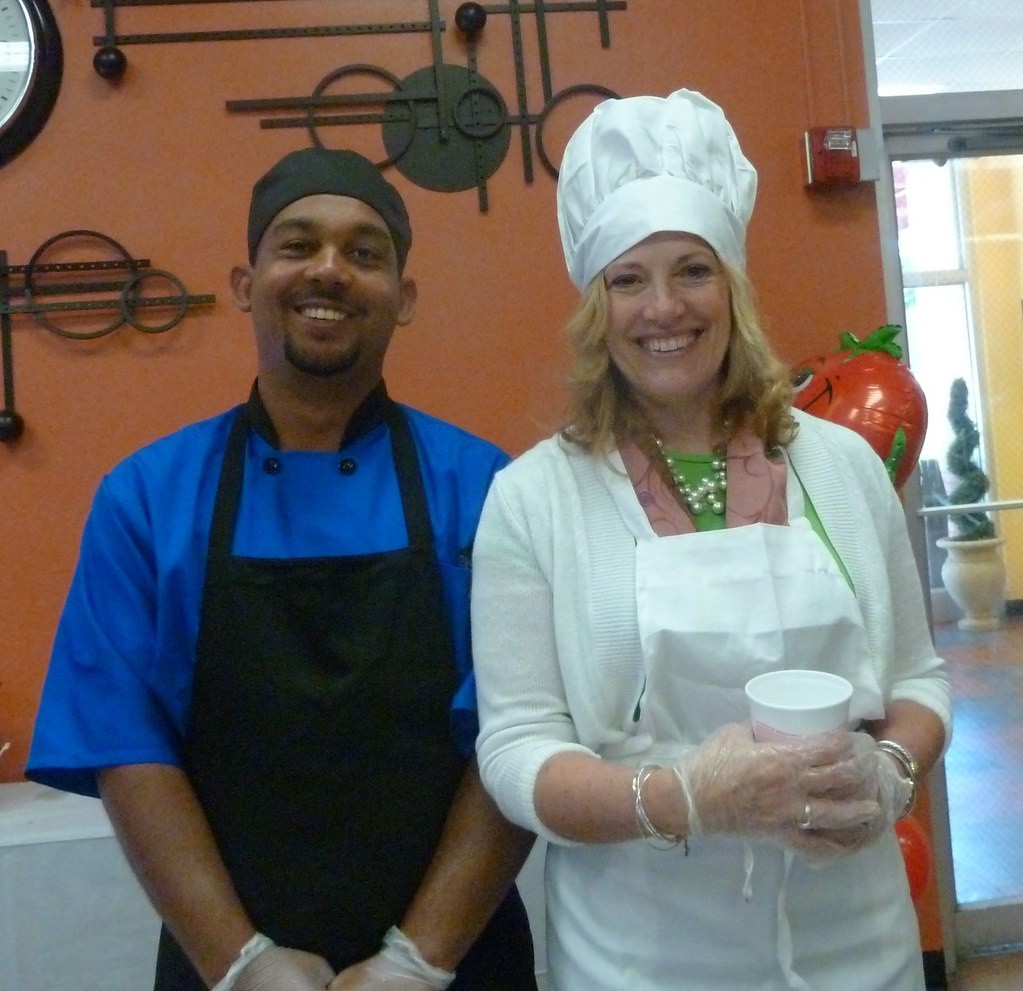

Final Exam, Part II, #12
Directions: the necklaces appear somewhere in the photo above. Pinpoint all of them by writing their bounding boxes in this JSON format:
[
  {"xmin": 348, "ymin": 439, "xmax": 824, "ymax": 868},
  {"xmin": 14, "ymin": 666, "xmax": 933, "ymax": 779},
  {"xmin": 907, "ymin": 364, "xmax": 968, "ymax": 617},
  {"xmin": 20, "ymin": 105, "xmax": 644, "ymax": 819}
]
[{"xmin": 649, "ymin": 418, "xmax": 734, "ymax": 521}]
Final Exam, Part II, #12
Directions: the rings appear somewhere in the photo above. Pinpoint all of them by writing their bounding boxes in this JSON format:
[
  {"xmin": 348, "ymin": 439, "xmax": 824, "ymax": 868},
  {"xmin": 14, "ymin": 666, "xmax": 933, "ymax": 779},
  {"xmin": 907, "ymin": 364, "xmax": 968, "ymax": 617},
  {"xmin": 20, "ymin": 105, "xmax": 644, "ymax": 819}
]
[{"xmin": 798, "ymin": 799, "xmax": 813, "ymax": 831}]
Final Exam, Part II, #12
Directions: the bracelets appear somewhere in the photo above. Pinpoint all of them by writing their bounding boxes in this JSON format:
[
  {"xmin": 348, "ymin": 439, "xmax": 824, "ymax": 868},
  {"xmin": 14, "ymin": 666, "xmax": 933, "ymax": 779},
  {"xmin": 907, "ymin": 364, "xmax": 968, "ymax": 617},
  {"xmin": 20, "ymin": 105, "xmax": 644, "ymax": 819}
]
[
  {"xmin": 878, "ymin": 739, "xmax": 922, "ymax": 821},
  {"xmin": 631, "ymin": 761, "xmax": 696, "ymax": 858}
]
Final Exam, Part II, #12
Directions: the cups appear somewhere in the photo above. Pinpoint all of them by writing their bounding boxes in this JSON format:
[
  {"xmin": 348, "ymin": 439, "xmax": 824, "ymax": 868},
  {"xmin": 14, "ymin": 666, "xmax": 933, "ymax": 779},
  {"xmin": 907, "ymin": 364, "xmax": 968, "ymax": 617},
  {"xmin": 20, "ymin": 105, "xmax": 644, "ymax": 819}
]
[{"xmin": 744, "ymin": 668, "xmax": 854, "ymax": 799}]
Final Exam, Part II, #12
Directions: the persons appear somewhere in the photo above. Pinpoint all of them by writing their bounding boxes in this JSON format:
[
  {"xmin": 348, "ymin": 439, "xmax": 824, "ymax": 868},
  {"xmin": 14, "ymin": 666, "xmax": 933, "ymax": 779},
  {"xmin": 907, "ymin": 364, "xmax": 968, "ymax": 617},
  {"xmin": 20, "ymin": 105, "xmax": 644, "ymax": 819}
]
[
  {"xmin": 17, "ymin": 145, "xmax": 546, "ymax": 990},
  {"xmin": 467, "ymin": 172, "xmax": 956, "ymax": 990}
]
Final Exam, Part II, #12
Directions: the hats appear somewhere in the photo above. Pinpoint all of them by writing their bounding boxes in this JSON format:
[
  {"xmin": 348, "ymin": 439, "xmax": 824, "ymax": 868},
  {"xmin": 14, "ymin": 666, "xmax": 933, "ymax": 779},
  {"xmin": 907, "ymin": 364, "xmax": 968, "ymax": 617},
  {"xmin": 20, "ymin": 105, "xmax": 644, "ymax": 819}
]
[
  {"xmin": 553, "ymin": 86, "xmax": 759, "ymax": 292},
  {"xmin": 246, "ymin": 147, "xmax": 413, "ymax": 285}
]
